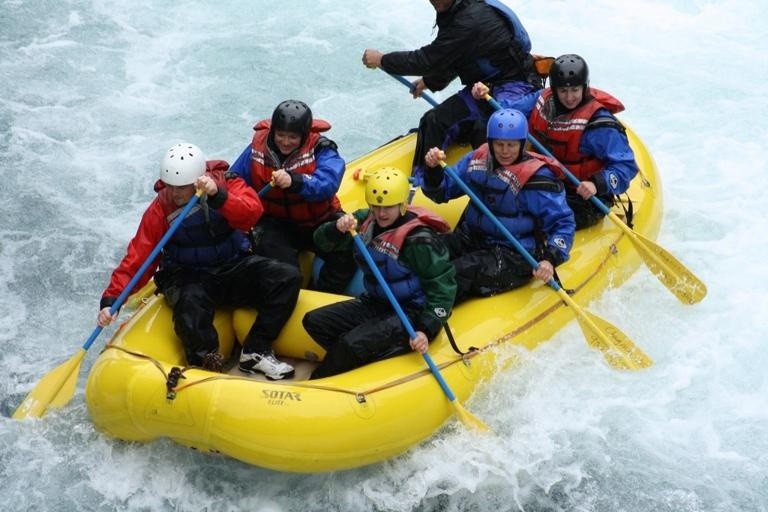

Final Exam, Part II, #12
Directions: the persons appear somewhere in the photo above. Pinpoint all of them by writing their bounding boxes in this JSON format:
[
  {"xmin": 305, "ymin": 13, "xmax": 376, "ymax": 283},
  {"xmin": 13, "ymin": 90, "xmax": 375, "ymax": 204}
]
[
  {"xmin": 472, "ymin": 54, "xmax": 639, "ymax": 232},
  {"xmin": 362, "ymin": 0, "xmax": 546, "ymax": 177},
  {"xmin": 301, "ymin": 166, "xmax": 456, "ymax": 381},
  {"xmin": 228, "ymin": 100, "xmax": 347, "ymax": 289},
  {"xmin": 416, "ymin": 110, "xmax": 576, "ymax": 296},
  {"xmin": 97, "ymin": 141, "xmax": 303, "ymax": 380}
]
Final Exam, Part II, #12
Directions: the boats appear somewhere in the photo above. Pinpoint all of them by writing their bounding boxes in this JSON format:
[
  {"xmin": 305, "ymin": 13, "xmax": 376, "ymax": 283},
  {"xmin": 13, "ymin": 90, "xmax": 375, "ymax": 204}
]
[{"xmin": 84, "ymin": 116, "xmax": 662, "ymax": 474}]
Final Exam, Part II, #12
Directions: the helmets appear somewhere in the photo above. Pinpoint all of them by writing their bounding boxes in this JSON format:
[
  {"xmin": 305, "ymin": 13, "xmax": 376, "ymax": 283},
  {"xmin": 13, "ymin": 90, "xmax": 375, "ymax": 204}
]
[
  {"xmin": 486, "ymin": 108, "xmax": 527, "ymax": 157},
  {"xmin": 161, "ymin": 142, "xmax": 206, "ymax": 187},
  {"xmin": 271, "ymin": 100, "xmax": 313, "ymax": 145},
  {"xmin": 364, "ymin": 167, "xmax": 410, "ymax": 216},
  {"xmin": 549, "ymin": 53, "xmax": 589, "ymax": 107}
]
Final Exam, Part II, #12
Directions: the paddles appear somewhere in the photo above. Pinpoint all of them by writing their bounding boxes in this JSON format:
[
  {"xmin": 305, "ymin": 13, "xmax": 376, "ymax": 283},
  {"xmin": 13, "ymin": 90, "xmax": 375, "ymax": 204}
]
[
  {"xmin": 343, "ymin": 218, "xmax": 492, "ymax": 432},
  {"xmin": 434, "ymin": 151, "xmax": 655, "ymax": 372},
  {"xmin": 476, "ymin": 85, "xmax": 707, "ymax": 304},
  {"xmin": 12, "ymin": 188, "xmax": 205, "ymax": 421}
]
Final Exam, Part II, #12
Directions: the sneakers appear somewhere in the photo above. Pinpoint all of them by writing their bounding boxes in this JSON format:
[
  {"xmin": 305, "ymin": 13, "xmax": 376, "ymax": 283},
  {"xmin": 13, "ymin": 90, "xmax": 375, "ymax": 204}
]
[
  {"xmin": 238, "ymin": 348, "xmax": 294, "ymax": 380},
  {"xmin": 204, "ymin": 352, "xmax": 223, "ymax": 372}
]
